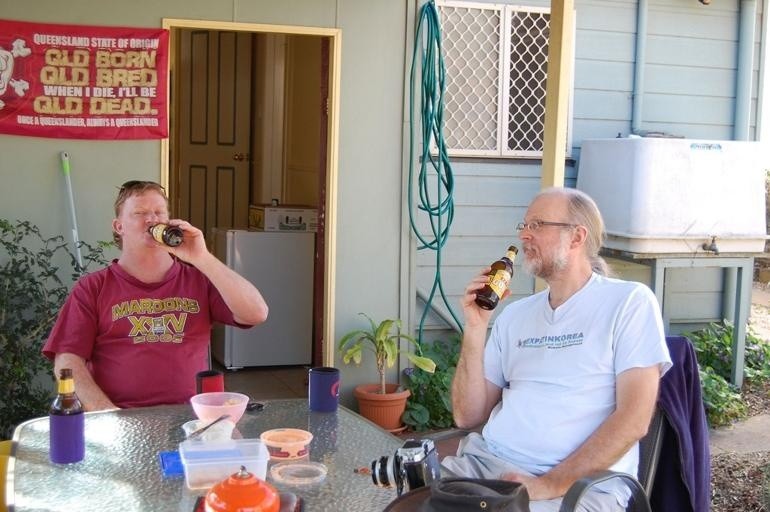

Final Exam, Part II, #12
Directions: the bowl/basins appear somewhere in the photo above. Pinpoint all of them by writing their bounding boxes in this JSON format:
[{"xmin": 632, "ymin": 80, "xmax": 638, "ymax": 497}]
[
  {"xmin": 190, "ymin": 391, "xmax": 249, "ymax": 423},
  {"xmin": 182, "ymin": 419, "xmax": 236, "ymax": 442}
]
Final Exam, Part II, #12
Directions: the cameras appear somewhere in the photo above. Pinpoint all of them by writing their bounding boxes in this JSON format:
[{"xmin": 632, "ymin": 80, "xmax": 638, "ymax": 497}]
[{"xmin": 371, "ymin": 437, "xmax": 441, "ymax": 491}]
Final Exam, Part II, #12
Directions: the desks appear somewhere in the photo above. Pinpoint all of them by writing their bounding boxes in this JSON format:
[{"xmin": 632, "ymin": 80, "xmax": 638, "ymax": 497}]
[
  {"xmin": 5, "ymin": 393, "xmax": 455, "ymax": 512},
  {"xmin": 596, "ymin": 246, "xmax": 770, "ymax": 396}
]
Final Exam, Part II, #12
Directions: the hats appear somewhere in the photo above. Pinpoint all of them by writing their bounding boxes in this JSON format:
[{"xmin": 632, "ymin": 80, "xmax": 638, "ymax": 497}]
[{"xmin": 382, "ymin": 476, "xmax": 530, "ymax": 512}]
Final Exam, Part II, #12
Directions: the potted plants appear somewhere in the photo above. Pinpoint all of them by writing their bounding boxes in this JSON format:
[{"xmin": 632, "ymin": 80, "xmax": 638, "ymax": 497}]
[{"xmin": 339, "ymin": 311, "xmax": 435, "ymax": 429}]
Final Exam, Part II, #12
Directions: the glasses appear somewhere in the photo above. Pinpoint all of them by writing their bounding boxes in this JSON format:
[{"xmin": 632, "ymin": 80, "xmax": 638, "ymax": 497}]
[
  {"xmin": 517, "ymin": 221, "xmax": 569, "ymax": 234},
  {"xmin": 118, "ymin": 180, "xmax": 166, "ymax": 197}
]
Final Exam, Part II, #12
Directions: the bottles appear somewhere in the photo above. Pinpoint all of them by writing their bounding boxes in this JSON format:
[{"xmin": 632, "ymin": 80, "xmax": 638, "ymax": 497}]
[
  {"xmin": 49, "ymin": 368, "xmax": 86, "ymax": 463},
  {"xmin": 474, "ymin": 245, "xmax": 519, "ymax": 310},
  {"xmin": 148, "ymin": 223, "xmax": 184, "ymax": 248}
]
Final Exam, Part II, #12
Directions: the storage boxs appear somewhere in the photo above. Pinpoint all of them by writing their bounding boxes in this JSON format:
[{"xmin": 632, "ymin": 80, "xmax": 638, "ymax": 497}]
[{"xmin": 250, "ymin": 204, "xmax": 318, "ymax": 232}]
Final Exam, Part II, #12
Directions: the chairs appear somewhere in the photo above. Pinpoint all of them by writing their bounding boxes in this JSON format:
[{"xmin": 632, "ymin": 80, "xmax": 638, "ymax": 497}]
[{"xmin": 423, "ymin": 337, "xmax": 710, "ymax": 512}]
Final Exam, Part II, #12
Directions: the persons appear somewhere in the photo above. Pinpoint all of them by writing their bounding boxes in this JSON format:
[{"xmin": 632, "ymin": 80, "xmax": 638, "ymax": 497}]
[
  {"xmin": 41, "ymin": 179, "xmax": 270, "ymax": 412},
  {"xmin": 440, "ymin": 186, "xmax": 675, "ymax": 512}
]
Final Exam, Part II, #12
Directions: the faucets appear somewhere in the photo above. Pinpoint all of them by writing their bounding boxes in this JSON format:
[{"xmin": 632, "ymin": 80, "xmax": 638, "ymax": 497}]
[{"xmin": 702, "ymin": 235, "xmax": 720, "ymax": 259}]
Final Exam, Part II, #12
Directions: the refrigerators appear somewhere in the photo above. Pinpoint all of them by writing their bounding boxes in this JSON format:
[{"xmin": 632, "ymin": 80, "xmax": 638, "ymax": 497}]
[{"xmin": 210, "ymin": 227, "xmax": 316, "ymax": 372}]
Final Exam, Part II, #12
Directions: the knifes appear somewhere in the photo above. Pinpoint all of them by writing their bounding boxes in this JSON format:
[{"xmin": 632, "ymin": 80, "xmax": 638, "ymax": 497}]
[{"xmin": 188, "ymin": 414, "xmax": 231, "ymax": 440}]
